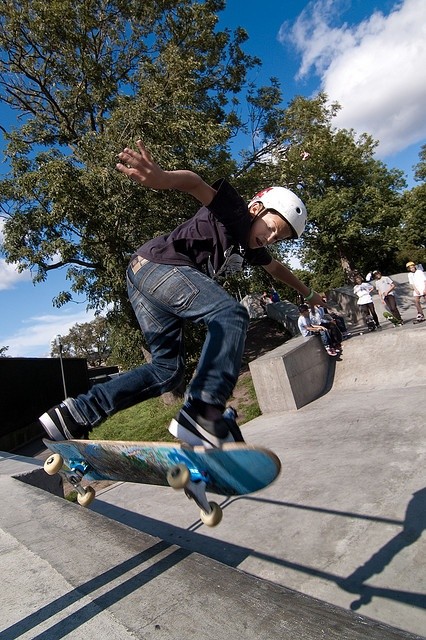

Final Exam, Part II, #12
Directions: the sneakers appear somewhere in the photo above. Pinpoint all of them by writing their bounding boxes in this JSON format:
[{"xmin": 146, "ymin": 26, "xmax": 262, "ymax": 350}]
[
  {"xmin": 416, "ymin": 314, "xmax": 424, "ymax": 319},
  {"xmin": 40, "ymin": 398, "xmax": 94, "ymax": 442},
  {"xmin": 167, "ymin": 404, "xmax": 244, "ymax": 447},
  {"xmin": 327, "ymin": 348, "xmax": 337, "ymax": 356}
]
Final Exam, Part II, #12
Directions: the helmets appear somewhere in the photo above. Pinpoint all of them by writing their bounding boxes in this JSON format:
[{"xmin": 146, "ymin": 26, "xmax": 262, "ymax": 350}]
[
  {"xmin": 247, "ymin": 187, "xmax": 308, "ymax": 239},
  {"xmin": 405, "ymin": 262, "xmax": 414, "ymax": 267}
]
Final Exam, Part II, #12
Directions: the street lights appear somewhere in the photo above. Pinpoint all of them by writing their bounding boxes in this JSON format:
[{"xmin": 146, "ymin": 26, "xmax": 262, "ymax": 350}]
[{"xmin": 55, "ymin": 335, "xmax": 69, "ymax": 400}]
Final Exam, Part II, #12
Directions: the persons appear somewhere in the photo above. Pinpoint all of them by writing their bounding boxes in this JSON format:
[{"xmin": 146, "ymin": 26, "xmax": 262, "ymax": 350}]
[
  {"xmin": 39, "ymin": 140, "xmax": 326, "ymax": 447},
  {"xmin": 354, "ymin": 275, "xmax": 382, "ymax": 332},
  {"xmin": 373, "ymin": 270, "xmax": 403, "ymax": 322},
  {"xmin": 298, "ymin": 306, "xmax": 337, "ymax": 356},
  {"xmin": 406, "ymin": 262, "xmax": 426, "ymax": 320},
  {"xmin": 322, "ymin": 300, "xmax": 347, "ymax": 333},
  {"xmin": 315, "ymin": 303, "xmax": 345, "ymax": 344},
  {"xmin": 308, "ymin": 303, "xmax": 341, "ymax": 353}
]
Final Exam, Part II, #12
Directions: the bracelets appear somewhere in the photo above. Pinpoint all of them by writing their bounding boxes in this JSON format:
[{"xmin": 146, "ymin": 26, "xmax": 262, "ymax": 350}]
[{"xmin": 305, "ymin": 289, "xmax": 314, "ymax": 301}]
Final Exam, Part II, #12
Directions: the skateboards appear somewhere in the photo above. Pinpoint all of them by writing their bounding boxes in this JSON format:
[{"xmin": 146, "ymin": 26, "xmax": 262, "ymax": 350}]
[
  {"xmin": 43, "ymin": 438, "xmax": 281, "ymax": 526},
  {"xmin": 412, "ymin": 319, "xmax": 425, "ymax": 324},
  {"xmin": 341, "ymin": 325, "xmax": 368, "ymax": 339},
  {"xmin": 383, "ymin": 312, "xmax": 403, "ymax": 327},
  {"xmin": 365, "ymin": 315, "xmax": 377, "ymax": 332}
]
